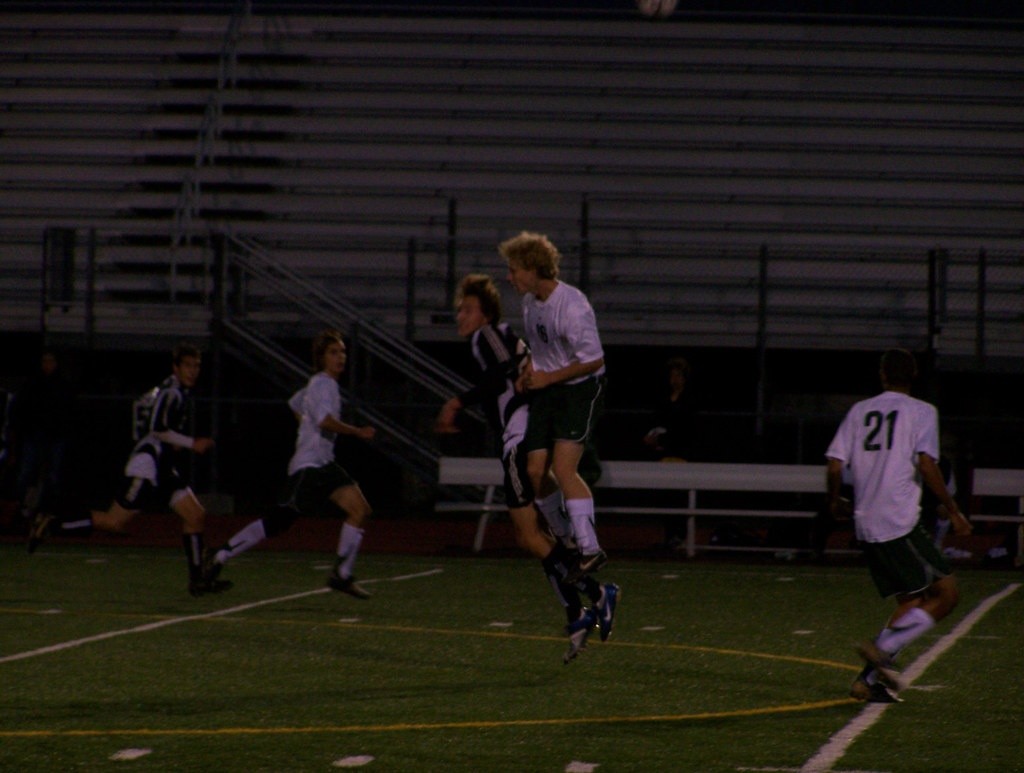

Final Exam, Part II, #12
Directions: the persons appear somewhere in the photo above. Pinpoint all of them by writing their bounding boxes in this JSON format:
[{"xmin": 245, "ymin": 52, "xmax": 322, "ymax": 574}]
[
  {"xmin": 498, "ymin": 230, "xmax": 609, "ymax": 573},
  {"xmin": 201, "ymin": 329, "xmax": 375, "ymax": 600},
  {"xmin": 454, "ymin": 274, "xmax": 622, "ymax": 665},
  {"xmin": 27, "ymin": 341, "xmax": 234, "ymax": 597},
  {"xmin": 825, "ymin": 348, "xmax": 972, "ymax": 703}
]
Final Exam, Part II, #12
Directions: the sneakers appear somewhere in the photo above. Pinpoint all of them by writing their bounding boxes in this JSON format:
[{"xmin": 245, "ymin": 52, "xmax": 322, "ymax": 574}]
[
  {"xmin": 552, "ymin": 541, "xmax": 580, "ymax": 559},
  {"xmin": 857, "ymin": 638, "xmax": 900, "ymax": 693},
  {"xmin": 188, "ymin": 573, "xmax": 233, "ymax": 597},
  {"xmin": 27, "ymin": 513, "xmax": 56, "ymax": 554},
  {"xmin": 591, "ymin": 583, "xmax": 622, "ymax": 642},
  {"xmin": 561, "ymin": 607, "xmax": 593, "ymax": 665},
  {"xmin": 199, "ymin": 547, "xmax": 222, "ymax": 593},
  {"xmin": 850, "ymin": 675, "xmax": 902, "ymax": 704},
  {"xmin": 562, "ymin": 550, "xmax": 608, "ymax": 585},
  {"xmin": 326, "ymin": 574, "xmax": 372, "ymax": 600}
]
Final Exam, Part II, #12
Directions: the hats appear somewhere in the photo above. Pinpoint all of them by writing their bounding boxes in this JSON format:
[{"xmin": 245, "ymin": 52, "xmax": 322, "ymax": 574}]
[{"xmin": 641, "ymin": 426, "xmax": 672, "ymax": 445}]
